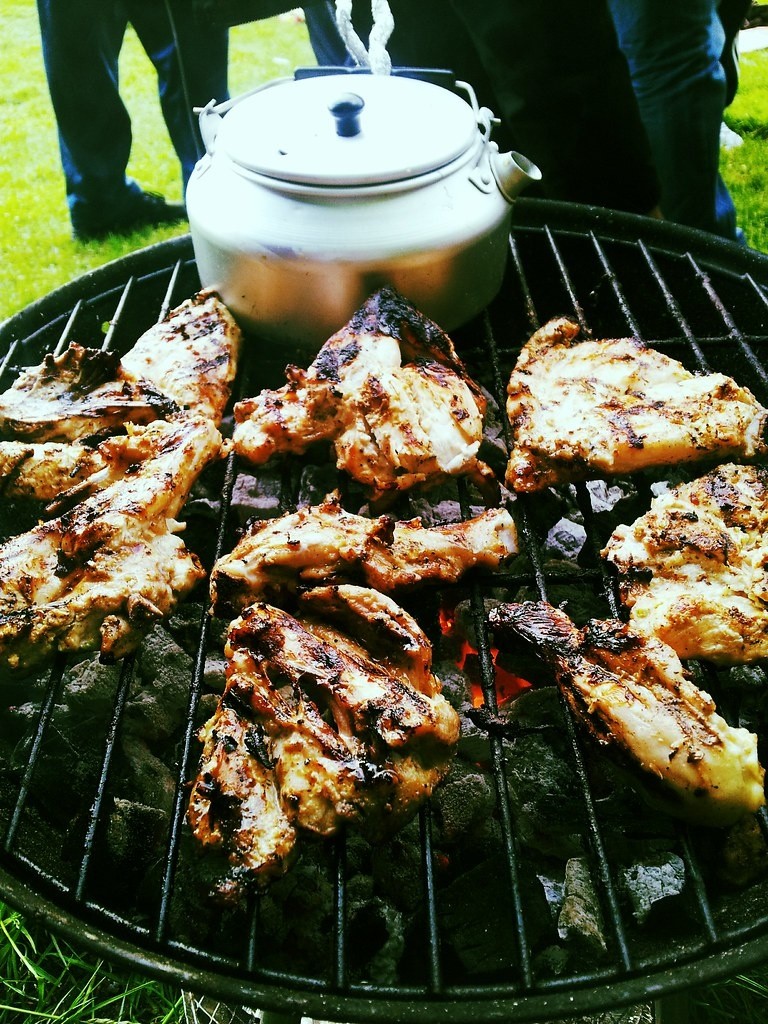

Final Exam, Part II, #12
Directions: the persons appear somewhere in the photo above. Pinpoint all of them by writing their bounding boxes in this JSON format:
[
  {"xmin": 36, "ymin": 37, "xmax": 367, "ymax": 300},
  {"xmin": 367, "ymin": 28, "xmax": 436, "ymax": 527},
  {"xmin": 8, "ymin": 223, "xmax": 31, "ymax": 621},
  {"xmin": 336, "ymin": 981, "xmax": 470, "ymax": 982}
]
[
  {"xmin": 36, "ymin": 0, "xmax": 229, "ymax": 240},
  {"xmin": 610, "ymin": 1, "xmax": 747, "ymax": 246}
]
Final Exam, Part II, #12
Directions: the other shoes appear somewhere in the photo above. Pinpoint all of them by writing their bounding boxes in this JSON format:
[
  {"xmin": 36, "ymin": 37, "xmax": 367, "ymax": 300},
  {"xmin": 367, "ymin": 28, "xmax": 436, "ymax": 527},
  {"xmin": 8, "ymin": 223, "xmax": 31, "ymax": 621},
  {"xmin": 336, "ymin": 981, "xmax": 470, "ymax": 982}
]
[{"xmin": 719, "ymin": 121, "xmax": 743, "ymax": 148}]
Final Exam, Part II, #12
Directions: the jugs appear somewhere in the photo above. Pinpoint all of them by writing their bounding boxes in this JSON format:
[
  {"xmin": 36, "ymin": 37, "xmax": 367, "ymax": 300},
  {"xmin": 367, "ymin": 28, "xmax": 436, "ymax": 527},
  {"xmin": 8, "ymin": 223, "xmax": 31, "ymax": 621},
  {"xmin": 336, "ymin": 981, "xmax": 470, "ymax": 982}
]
[{"xmin": 183, "ymin": 67, "xmax": 543, "ymax": 351}]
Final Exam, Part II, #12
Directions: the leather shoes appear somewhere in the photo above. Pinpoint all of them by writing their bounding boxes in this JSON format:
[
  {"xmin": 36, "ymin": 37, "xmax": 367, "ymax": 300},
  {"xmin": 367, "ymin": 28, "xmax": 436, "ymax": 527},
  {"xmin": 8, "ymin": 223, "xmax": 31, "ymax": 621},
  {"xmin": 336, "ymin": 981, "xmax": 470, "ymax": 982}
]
[{"xmin": 72, "ymin": 191, "xmax": 185, "ymax": 243}]
